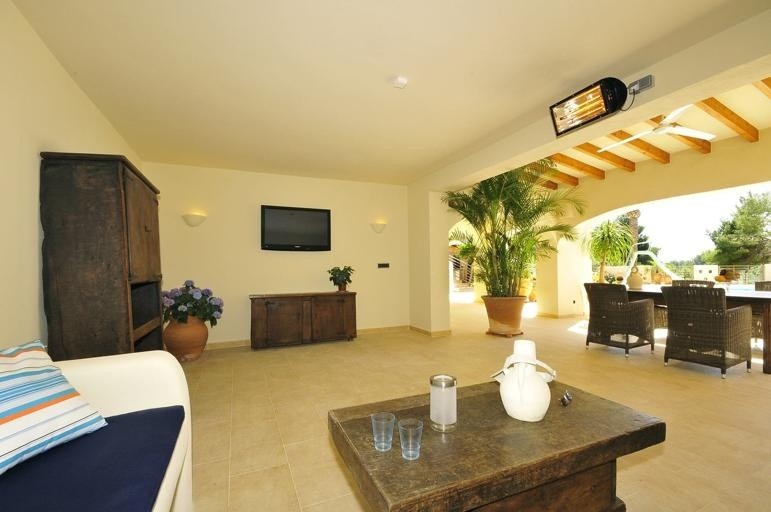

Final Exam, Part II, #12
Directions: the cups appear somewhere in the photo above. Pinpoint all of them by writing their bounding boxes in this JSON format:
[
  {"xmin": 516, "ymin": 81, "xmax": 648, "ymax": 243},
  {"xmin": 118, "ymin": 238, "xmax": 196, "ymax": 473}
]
[
  {"xmin": 397, "ymin": 418, "xmax": 424, "ymax": 459},
  {"xmin": 370, "ymin": 411, "xmax": 396, "ymax": 451},
  {"xmin": 429, "ymin": 373, "xmax": 459, "ymax": 432}
]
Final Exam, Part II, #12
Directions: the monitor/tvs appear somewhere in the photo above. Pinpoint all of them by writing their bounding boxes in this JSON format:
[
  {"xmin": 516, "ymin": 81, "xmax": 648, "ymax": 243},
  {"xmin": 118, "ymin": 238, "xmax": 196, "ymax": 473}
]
[{"xmin": 260, "ymin": 204, "xmax": 331, "ymax": 251}]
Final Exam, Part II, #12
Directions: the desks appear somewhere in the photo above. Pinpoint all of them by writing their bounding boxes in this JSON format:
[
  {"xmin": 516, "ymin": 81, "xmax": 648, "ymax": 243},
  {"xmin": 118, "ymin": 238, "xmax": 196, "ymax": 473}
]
[{"xmin": 620, "ymin": 285, "xmax": 771, "ymax": 374}]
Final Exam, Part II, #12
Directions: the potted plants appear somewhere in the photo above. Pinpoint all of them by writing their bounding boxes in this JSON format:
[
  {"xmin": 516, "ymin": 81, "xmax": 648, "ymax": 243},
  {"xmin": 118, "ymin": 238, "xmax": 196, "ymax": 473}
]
[
  {"xmin": 326, "ymin": 266, "xmax": 354, "ymax": 291},
  {"xmin": 440, "ymin": 157, "xmax": 587, "ymax": 339}
]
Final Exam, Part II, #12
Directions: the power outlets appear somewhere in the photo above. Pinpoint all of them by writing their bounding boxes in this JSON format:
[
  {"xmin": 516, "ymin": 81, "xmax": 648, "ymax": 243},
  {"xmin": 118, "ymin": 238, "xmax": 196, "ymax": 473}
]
[{"xmin": 628, "ymin": 82, "xmax": 638, "ymax": 93}]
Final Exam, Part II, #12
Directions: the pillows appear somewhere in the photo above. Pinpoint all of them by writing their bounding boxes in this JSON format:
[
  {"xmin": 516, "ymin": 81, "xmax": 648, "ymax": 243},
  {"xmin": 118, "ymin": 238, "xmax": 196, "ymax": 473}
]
[{"xmin": 0, "ymin": 336, "xmax": 108, "ymax": 479}]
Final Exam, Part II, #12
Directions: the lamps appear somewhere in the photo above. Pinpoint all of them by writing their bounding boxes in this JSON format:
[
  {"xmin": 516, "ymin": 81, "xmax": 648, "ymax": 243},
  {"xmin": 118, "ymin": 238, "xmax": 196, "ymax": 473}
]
[
  {"xmin": 549, "ymin": 77, "xmax": 629, "ymax": 137},
  {"xmin": 180, "ymin": 212, "xmax": 207, "ymax": 227},
  {"xmin": 371, "ymin": 222, "xmax": 387, "ymax": 234}
]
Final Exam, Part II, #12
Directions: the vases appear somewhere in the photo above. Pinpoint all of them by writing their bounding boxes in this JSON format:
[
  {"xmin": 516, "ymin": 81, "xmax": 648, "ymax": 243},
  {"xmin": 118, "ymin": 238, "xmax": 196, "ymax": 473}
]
[{"xmin": 164, "ymin": 312, "xmax": 209, "ymax": 364}]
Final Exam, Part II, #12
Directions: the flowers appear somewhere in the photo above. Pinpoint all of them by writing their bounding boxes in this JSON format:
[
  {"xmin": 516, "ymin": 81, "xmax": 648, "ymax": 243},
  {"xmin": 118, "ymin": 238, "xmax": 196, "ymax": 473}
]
[{"xmin": 161, "ymin": 278, "xmax": 225, "ymax": 326}]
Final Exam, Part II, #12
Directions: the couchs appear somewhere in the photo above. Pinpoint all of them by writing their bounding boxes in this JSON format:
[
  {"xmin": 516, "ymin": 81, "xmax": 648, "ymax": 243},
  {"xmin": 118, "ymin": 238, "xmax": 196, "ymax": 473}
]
[{"xmin": 0, "ymin": 349, "xmax": 201, "ymax": 511}]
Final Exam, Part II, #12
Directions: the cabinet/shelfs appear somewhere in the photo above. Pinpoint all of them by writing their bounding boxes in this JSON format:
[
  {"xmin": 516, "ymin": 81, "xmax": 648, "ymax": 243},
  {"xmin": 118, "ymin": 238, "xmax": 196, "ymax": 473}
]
[
  {"xmin": 250, "ymin": 291, "xmax": 358, "ymax": 351},
  {"xmin": 38, "ymin": 151, "xmax": 165, "ymax": 358}
]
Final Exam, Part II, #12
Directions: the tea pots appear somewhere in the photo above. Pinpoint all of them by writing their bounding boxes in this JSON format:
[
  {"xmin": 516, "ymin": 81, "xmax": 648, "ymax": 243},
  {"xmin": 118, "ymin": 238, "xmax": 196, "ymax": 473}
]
[
  {"xmin": 627, "ymin": 266, "xmax": 643, "ymax": 290},
  {"xmin": 491, "ymin": 338, "xmax": 557, "ymax": 422}
]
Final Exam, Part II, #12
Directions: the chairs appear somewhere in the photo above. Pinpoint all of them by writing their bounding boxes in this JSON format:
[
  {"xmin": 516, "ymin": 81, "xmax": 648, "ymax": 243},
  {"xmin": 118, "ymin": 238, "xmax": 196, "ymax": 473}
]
[
  {"xmin": 660, "ymin": 285, "xmax": 753, "ymax": 380},
  {"xmin": 653, "ymin": 279, "xmax": 716, "ymax": 330},
  {"xmin": 583, "ymin": 280, "xmax": 656, "ymax": 357},
  {"xmin": 748, "ymin": 280, "xmax": 771, "ymax": 343}
]
